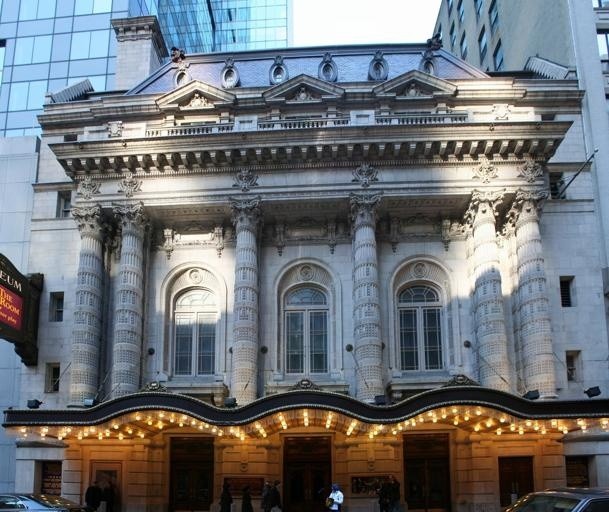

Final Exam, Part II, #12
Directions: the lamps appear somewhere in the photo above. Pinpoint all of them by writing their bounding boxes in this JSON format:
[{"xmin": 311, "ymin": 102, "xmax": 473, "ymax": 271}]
[
  {"xmin": 224, "ymin": 396, "xmax": 238, "ymax": 409},
  {"xmin": 84, "ymin": 398, "xmax": 99, "ymax": 408},
  {"xmin": 374, "ymin": 395, "xmax": 386, "ymax": 406},
  {"xmin": 522, "ymin": 389, "xmax": 539, "ymax": 400},
  {"xmin": 584, "ymin": 386, "xmax": 601, "ymax": 398},
  {"xmin": 27, "ymin": 399, "xmax": 39, "ymax": 409}
]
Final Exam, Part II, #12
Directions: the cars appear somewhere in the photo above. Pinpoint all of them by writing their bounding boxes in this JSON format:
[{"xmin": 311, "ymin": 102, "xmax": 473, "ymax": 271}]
[
  {"xmin": 505, "ymin": 483, "xmax": 609, "ymax": 512},
  {"xmin": 0, "ymin": 492, "xmax": 89, "ymax": 512}
]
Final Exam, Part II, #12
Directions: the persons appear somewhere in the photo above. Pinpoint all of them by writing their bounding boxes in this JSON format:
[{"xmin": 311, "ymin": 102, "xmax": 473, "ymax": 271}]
[
  {"xmin": 241, "ymin": 485, "xmax": 254, "ymax": 512},
  {"xmin": 389, "ymin": 474, "xmax": 400, "ymax": 511},
  {"xmin": 103, "ymin": 480, "xmax": 122, "ymax": 511},
  {"xmin": 326, "ymin": 484, "xmax": 344, "ymax": 512},
  {"xmin": 219, "ymin": 484, "xmax": 233, "ymax": 512},
  {"xmin": 85, "ymin": 481, "xmax": 102, "ymax": 512},
  {"xmin": 378, "ymin": 475, "xmax": 392, "ymax": 512},
  {"xmin": 261, "ymin": 481, "xmax": 274, "ymax": 512},
  {"xmin": 269, "ymin": 480, "xmax": 283, "ymax": 512}
]
